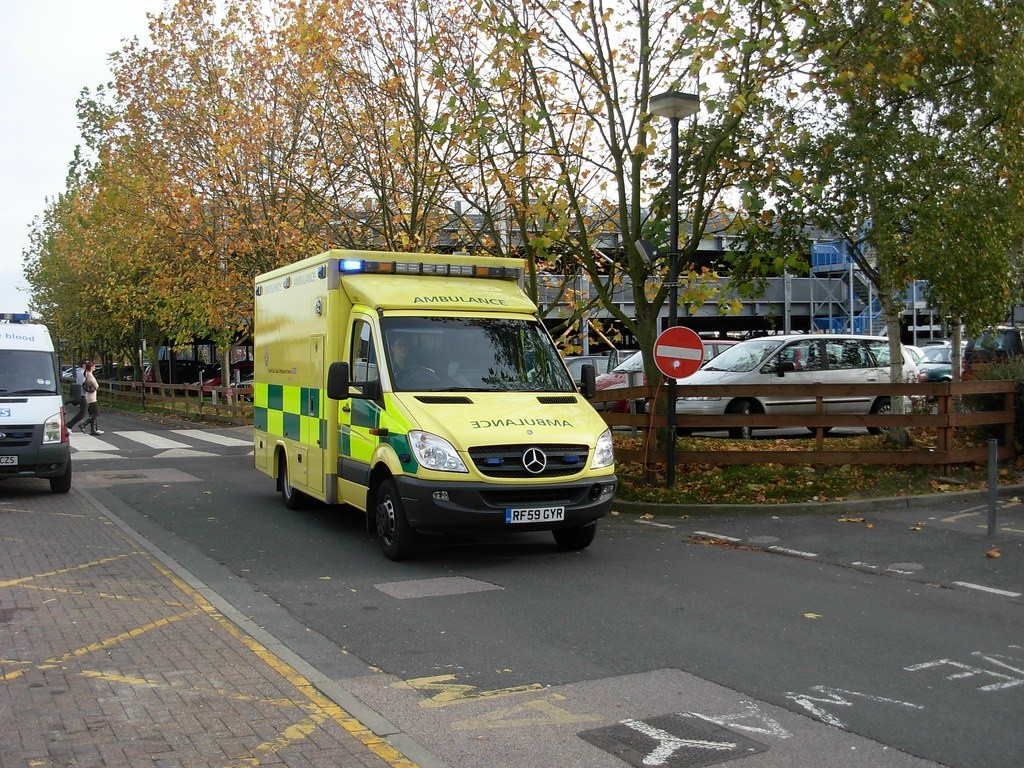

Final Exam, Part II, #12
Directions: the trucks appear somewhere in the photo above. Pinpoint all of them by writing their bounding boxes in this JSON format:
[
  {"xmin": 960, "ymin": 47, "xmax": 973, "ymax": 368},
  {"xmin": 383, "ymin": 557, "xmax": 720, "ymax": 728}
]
[{"xmin": 0, "ymin": 312, "xmax": 81, "ymax": 494}]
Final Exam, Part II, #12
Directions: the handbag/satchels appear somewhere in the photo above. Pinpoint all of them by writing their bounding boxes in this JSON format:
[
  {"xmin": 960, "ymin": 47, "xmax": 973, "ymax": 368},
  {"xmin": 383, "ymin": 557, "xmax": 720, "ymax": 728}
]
[{"xmin": 82, "ymin": 380, "xmax": 96, "ymax": 393}]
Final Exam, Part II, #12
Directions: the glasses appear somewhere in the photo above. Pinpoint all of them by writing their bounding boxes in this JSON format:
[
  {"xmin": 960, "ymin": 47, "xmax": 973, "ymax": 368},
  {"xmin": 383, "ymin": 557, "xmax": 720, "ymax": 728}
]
[{"xmin": 391, "ymin": 342, "xmax": 409, "ymax": 348}]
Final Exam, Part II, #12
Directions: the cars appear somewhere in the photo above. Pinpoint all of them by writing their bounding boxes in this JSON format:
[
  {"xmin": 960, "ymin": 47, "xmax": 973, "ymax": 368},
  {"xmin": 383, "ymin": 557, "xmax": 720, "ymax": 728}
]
[
  {"xmin": 59, "ymin": 359, "xmax": 253, "ymax": 401},
  {"xmin": 645, "ymin": 333, "xmax": 916, "ymax": 439},
  {"xmin": 581, "ymin": 336, "xmax": 972, "ymax": 405},
  {"xmin": 578, "ymin": 339, "xmax": 745, "ymax": 430},
  {"xmin": 963, "ymin": 323, "xmax": 1024, "ymax": 449},
  {"xmin": 516, "ymin": 355, "xmax": 623, "ymax": 393}
]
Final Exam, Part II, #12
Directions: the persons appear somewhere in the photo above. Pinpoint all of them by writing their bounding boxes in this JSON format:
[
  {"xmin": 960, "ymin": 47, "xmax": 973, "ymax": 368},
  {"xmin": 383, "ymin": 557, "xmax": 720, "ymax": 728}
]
[
  {"xmin": 78, "ymin": 361, "xmax": 103, "ymax": 436},
  {"xmin": 65, "ymin": 359, "xmax": 105, "ymax": 435},
  {"xmin": 389, "ymin": 332, "xmax": 414, "ymax": 379}
]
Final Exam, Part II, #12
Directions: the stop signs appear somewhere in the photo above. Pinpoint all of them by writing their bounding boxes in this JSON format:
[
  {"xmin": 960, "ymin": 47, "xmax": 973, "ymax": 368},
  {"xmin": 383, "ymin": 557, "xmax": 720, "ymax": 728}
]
[{"xmin": 653, "ymin": 326, "xmax": 705, "ymax": 381}]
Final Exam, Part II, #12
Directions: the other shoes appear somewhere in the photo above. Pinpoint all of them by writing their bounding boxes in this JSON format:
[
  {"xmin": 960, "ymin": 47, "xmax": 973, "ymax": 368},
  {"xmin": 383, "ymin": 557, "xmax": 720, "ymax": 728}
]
[
  {"xmin": 91, "ymin": 430, "xmax": 105, "ymax": 436},
  {"xmin": 66, "ymin": 426, "xmax": 73, "ymax": 435},
  {"xmin": 79, "ymin": 424, "xmax": 86, "ymax": 434}
]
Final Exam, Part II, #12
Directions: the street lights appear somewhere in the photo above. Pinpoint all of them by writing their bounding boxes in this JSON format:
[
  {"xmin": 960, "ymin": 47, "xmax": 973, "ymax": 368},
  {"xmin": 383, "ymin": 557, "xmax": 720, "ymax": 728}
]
[{"xmin": 650, "ymin": 89, "xmax": 700, "ymax": 485}]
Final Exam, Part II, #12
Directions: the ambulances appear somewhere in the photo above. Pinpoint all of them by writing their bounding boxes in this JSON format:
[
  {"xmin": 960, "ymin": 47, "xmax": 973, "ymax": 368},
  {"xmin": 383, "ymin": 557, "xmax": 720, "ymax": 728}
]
[{"xmin": 247, "ymin": 249, "xmax": 618, "ymax": 561}]
